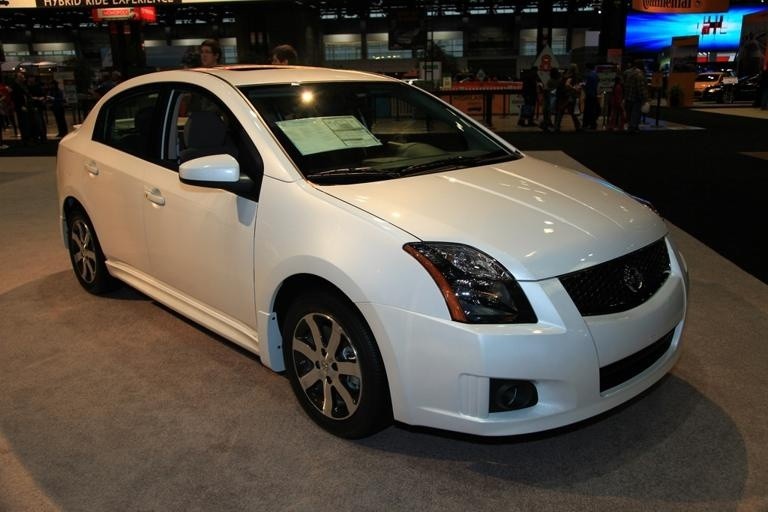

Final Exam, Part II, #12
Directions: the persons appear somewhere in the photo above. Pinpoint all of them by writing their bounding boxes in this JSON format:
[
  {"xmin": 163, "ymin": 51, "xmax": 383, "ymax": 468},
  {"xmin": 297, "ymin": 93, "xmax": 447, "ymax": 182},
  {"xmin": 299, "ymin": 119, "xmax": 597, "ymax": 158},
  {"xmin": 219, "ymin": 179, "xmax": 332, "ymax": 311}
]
[
  {"xmin": 0, "ymin": 72, "xmax": 68, "ymax": 149},
  {"xmin": 198, "ymin": 39, "xmax": 222, "ymax": 67},
  {"xmin": 517, "ymin": 59, "xmax": 648, "ymax": 135},
  {"xmin": 271, "ymin": 45, "xmax": 298, "ymax": 65}
]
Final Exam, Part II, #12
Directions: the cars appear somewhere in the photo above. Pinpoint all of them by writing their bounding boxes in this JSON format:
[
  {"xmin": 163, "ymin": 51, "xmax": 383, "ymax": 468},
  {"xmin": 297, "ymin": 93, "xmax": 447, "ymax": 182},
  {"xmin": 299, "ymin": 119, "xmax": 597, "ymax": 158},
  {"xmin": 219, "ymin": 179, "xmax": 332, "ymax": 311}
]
[
  {"xmin": 55, "ymin": 64, "xmax": 692, "ymax": 439},
  {"xmin": 694, "ymin": 68, "xmax": 768, "ymax": 103},
  {"xmin": 11, "ymin": 60, "xmax": 78, "ymax": 106},
  {"xmin": 593, "ymin": 58, "xmax": 671, "ymax": 94}
]
